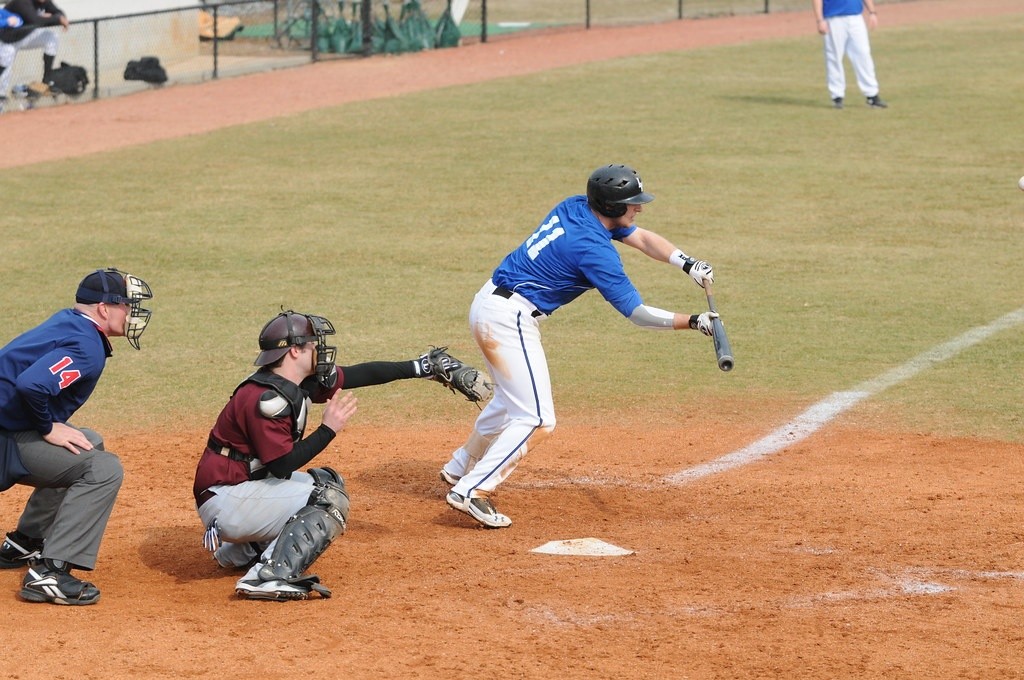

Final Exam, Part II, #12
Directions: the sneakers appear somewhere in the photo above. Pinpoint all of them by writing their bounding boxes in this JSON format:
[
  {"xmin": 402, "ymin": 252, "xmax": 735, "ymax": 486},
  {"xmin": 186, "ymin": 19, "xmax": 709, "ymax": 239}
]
[
  {"xmin": 447, "ymin": 491, "xmax": 512, "ymax": 529},
  {"xmin": 439, "ymin": 458, "xmax": 466, "ymax": 486},
  {"xmin": 0, "ymin": 531, "xmax": 44, "ymax": 568},
  {"xmin": 235, "ymin": 562, "xmax": 309, "ymax": 601},
  {"xmin": 20, "ymin": 563, "xmax": 100, "ymax": 605},
  {"xmin": 213, "ymin": 542, "xmax": 268, "ymax": 569}
]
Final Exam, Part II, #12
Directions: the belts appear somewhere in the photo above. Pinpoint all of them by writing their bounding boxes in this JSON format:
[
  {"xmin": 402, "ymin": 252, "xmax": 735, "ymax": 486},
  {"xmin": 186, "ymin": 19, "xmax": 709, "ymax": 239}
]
[
  {"xmin": 196, "ymin": 490, "xmax": 217, "ymax": 509},
  {"xmin": 493, "ymin": 287, "xmax": 542, "ymax": 317}
]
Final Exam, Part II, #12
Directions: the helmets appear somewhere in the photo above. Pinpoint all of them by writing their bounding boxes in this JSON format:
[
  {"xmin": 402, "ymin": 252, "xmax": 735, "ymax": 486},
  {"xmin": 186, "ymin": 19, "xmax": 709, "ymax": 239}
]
[
  {"xmin": 253, "ymin": 312, "xmax": 316, "ymax": 366},
  {"xmin": 587, "ymin": 164, "xmax": 655, "ymax": 217}
]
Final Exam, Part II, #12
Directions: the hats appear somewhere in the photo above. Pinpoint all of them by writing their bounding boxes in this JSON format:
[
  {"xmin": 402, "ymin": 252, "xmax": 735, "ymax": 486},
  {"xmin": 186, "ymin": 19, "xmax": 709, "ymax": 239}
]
[{"xmin": 76, "ymin": 269, "xmax": 127, "ymax": 304}]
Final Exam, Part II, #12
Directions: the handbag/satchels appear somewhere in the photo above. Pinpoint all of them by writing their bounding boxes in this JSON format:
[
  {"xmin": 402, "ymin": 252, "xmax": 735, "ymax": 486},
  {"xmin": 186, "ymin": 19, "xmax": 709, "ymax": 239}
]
[
  {"xmin": 124, "ymin": 57, "xmax": 167, "ymax": 82},
  {"xmin": 50, "ymin": 62, "xmax": 88, "ymax": 93}
]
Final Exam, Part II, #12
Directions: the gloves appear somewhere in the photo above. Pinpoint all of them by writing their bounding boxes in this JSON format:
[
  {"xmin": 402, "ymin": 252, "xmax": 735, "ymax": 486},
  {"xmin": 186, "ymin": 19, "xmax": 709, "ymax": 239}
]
[
  {"xmin": 698, "ymin": 312, "xmax": 723, "ymax": 336},
  {"xmin": 202, "ymin": 517, "xmax": 221, "ymax": 552},
  {"xmin": 689, "ymin": 260, "xmax": 714, "ymax": 288}
]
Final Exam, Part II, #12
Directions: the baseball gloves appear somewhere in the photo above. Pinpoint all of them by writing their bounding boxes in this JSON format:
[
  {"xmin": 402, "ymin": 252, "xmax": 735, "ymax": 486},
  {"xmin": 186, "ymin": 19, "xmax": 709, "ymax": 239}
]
[{"xmin": 418, "ymin": 344, "xmax": 499, "ymax": 413}]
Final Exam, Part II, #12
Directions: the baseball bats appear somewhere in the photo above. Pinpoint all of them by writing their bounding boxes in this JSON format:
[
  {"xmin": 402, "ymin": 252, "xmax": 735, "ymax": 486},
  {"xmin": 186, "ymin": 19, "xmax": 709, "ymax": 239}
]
[{"xmin": 703, "ymin": 279, "xmax": 735, "ymax": 372}]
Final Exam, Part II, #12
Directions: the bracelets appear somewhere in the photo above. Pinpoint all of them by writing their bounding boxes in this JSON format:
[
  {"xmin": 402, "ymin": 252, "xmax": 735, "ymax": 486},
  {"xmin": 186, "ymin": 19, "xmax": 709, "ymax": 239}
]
[{"xmin": 870, "ymin": 11, "xmax": 876, "ymax": 13}]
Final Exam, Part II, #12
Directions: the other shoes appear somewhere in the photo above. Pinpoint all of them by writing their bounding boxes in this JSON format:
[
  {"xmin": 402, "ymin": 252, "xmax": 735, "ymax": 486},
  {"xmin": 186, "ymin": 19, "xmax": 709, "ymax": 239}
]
[
  {"xmin": 833, "ymin": 97, "xmax": 842, "ymax": 108},
  {"xmin": 868, "ymin": 96, "xmax": 889, "ymax": 109}
]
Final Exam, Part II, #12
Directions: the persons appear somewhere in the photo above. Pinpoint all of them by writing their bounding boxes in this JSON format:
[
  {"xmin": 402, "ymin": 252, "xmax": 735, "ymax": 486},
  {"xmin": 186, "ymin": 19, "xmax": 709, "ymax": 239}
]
[
  {"xmin": 439, "ymin": 165, "xmax": 722, "ymax": 526},
  {"xmin": 0, "ymin": 8, "xmax": 24, "ymax": 28},
  {"xmin": 193, "ymin": 309, "xmax": 492, "ymax": 601},
  {"xmin": 0, "ymin": 0, "xmax": 69, "ymax": 100},
  {"xmin": 812, "ymin": 0, "xmax": 887, "ymax": 108},
  {"xmin": 1, "ymin": 266, "xmax": 154, "ymax": 606}
]
